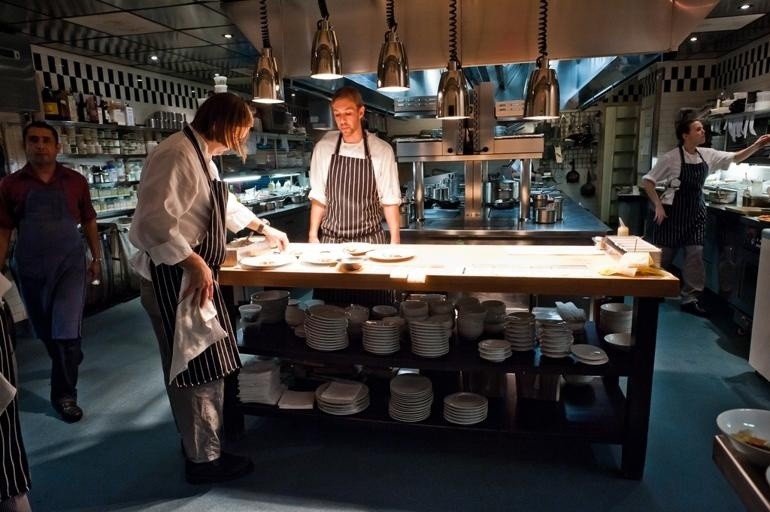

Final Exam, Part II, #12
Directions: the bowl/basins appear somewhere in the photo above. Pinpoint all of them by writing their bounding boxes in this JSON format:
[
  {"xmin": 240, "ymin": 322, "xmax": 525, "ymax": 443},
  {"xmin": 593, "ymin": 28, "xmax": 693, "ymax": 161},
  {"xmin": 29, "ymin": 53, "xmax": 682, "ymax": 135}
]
[
  {"xmin": 599, "ymin": 301, "xmax": 637, "ymax": 356},
  {"xmin": 346, "ymin": 305, "xmax": 405, "ymax": 334},
  {"xmin": 266, "ymin": 203, "xmax": 275, "ymax": 210},
  {"xmin": 250, "ymin": 291, "xmax": 290, "ymax": 325},
  {"xmin": 238, "ymin": 304, "xmax": 263, "ymax": 335},
  {"xmin": 453, "ymin": 296, "xmax": 484, "ymax": 339},
  {"xmin": 480, "ymin": 299, "xmax": 506, "ymax": 333},
  {"xmin": 715, "ymin": 408, "xmax": 770, "ymax": 460},
  {"xmin": 468, "ymin": 373, "xmax": 600, "ymax": 403},
  {"xmin": 401, "ymin": 297, "xmax": 455, "ymax": 339},
  {"xmin": 284, "ymin": 298, "xmax": 324, "ymax": 339}
]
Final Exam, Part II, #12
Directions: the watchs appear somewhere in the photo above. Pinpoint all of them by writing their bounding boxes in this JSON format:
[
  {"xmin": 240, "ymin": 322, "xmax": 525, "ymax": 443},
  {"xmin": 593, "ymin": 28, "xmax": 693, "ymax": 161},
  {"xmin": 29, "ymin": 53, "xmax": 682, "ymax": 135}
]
[{"xmin": 257, "ymin": 218, "xmax": 271, "ymax": 234}]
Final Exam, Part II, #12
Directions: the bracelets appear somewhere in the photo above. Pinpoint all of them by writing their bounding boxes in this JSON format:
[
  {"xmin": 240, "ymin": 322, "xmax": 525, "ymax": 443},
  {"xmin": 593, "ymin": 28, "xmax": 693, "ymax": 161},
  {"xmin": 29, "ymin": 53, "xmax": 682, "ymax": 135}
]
[{"xmin": 91, "ymin": 257, "xmax": 102, "ymax": 263}]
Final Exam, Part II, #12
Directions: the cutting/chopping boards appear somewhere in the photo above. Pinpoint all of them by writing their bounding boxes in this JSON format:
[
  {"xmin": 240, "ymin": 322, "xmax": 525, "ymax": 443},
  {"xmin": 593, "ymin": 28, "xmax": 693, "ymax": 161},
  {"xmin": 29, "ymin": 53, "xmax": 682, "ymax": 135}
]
[{"xmin": 725, "ymin": 205, "xmax": 770, "ymax": 216}]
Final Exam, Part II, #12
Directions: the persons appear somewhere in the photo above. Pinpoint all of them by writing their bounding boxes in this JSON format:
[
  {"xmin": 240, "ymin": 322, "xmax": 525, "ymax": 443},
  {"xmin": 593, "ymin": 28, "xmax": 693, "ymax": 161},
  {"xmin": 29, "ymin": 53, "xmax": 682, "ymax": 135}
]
[
  {"xmin": 641, "ymin": 120, "xmax": 770, "ymax": 317},
  {"xmin": 307, "ymin": 86, "xmax": 402, "ymax": 309},
  {"xmin": 0, "ymin": 120, "xmax": 102, "ymax": 424},
  {"xmin": 0, "ymin": 273, "xmax": 33, "ymax": 512},
  {"xmin": 129, "ymin": 93, "xmax": 291, "ymax": 484}
]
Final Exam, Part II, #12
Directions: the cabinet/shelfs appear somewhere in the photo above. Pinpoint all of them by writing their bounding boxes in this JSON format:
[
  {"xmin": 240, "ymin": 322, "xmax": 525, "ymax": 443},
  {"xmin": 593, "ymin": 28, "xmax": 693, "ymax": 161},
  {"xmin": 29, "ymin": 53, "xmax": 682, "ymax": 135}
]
[
  {"xmin": 44, "ymin": 118, "xmax": 311, "ymax": 222},
  {"xmin": 623, "ymin": 189, "xmax": 762, "ymax": 348}
]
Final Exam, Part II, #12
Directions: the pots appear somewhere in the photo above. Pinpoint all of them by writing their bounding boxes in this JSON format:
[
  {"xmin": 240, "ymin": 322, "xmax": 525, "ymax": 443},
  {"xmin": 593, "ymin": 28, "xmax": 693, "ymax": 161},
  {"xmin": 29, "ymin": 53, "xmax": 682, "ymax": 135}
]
[
  {"xmin": 497, "ymin": 179, "xmax": 564, "ymax": 224},
  {"xmin": 494, "ymin": 121, "xmax": 525, "ymax": 136},
  {"xmin": 291, "ymin": 196, "xmax": 305, "ymax": 203},
  {"xmin": 709, "ymin": 187, "xmax": 736, "ymax": 204},
  {"xmin": 566, "ymin": 157, "xmax": 597, "ymax": 199}
]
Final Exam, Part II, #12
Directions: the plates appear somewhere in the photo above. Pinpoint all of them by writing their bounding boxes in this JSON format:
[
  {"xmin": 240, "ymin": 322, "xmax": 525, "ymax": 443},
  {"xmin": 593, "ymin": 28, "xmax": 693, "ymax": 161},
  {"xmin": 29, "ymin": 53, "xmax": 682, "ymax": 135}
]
[
  {"xmin": 535, "ymin": 319, "xmax": 575, "ymax": 359},
  {"xmin": 360, "ymin": 320, "xmax": 402, "ymax": 353},
  {"xmin": 477, "ymin": 339, "xmax": 513, "ymax": 362},
  {"xmin": 568, "ymin": 343, "xmax": 606, "ymax": 365},
  {"xmin": 442, "ymin": 393, "xmax": 488, "ymax": 424},
  {"xmin": 388, "ymin": 374, "xmax": 434, "ymax": 422},
  {"xmin": 314, "ymin": 380, "xmax": 371, "ymax": 416},
  {"xmin": 303, "ymin": 303, "xmax": 350, "ymax": 350},
  {"xmin": 239, "ymin": 242, "xmax": 414, "ymax": 267},
  {"xmin": 410, "ymin": 320, "xmax": 449, "ymax": 357},
  {"xmin": 505, "ymin": 311, "xmax": 538, "ymax": 352}
]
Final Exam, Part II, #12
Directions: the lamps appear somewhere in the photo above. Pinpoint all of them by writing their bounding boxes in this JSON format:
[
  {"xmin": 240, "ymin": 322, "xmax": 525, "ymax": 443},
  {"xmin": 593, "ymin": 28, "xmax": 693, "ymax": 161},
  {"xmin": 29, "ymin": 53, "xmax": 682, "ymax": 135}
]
[
  {"xmin": 309, "ymin": 1, "xmax": 343, "ymax": 80},
  {"xmin": 253, "ymin": 1, "xmax": 286, "ymax": 107},
  {"xmin": 376, "ymin": 1, "xmax": 411, "ymax": 93},
  {"xmin": 434, "ymin": 3, "xmax": 471, "ymax": 120},
  {"xmin": 522, "ymin": 2, "xmax": 561, "ymax": 122}
]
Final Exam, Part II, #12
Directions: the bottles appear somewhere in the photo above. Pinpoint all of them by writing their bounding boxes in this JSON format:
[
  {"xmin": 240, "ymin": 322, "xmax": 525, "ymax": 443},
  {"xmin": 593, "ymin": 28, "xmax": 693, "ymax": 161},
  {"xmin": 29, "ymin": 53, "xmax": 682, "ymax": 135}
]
[
  {"xmin": 41, "ymin": 86, "xmax": 111, "ymax": 124},
  {"xmin": 269, "ymin": 180, "xmax": 281, "ymax": 191}
]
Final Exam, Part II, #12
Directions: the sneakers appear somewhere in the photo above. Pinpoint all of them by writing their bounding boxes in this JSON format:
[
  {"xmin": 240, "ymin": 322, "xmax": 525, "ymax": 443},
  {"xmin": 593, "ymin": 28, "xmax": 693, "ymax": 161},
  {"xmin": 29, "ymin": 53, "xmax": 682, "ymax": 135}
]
[
  {"xmin": 55, "ymin": 397, "xmax": 83, "ymax": 421},
  {"xmin": 181, "ymin": 437, "xmax": 253, "ymax": 486},
  {"xmin": 680, "ymin": 301, "xmax": 708, "ymax": 318}
]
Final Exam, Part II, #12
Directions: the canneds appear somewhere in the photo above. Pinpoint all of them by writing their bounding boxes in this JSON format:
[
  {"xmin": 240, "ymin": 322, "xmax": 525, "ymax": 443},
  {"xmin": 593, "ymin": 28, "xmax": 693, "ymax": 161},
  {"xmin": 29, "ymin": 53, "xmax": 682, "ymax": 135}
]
[{"xmin": 150, "ymin": 110, "xmax": 184, "ymax": 131}]
